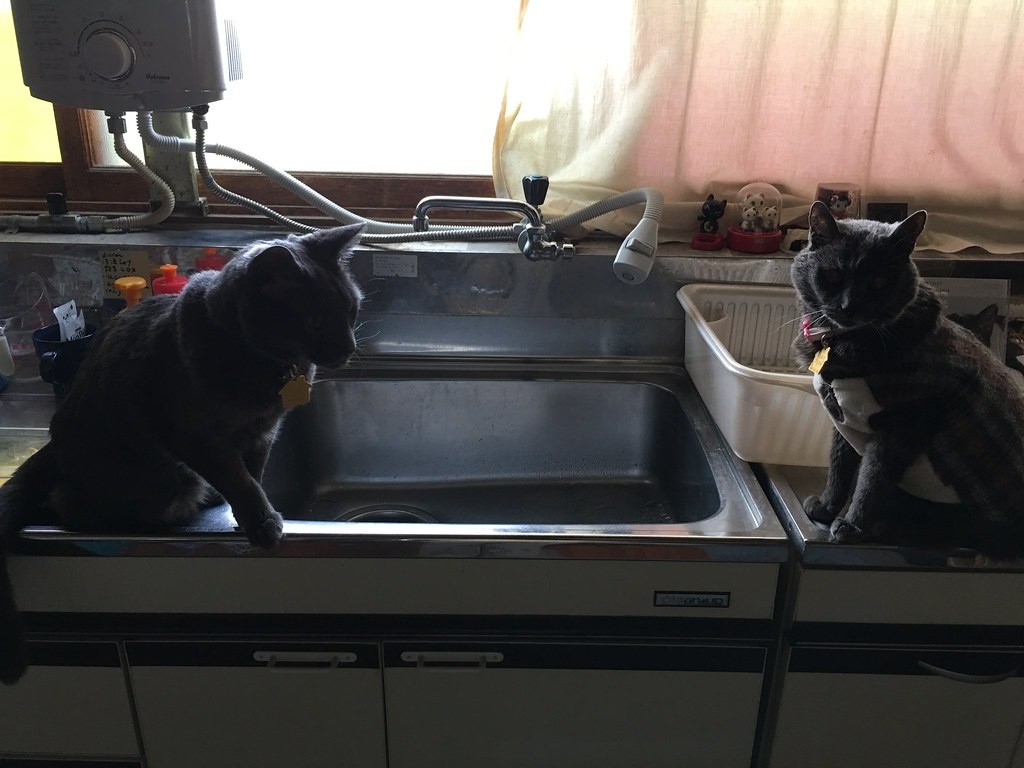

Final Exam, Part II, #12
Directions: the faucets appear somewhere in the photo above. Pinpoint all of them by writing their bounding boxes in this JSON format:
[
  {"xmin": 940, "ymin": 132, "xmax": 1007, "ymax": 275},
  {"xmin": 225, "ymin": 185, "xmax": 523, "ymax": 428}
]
[{"xmin": 411, "ymin": 195, "xmax": 550, "ymax": 260}]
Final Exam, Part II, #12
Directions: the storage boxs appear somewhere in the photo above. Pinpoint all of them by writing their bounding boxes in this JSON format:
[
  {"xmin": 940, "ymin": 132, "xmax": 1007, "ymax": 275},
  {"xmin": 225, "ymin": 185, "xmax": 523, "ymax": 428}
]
[{"xmin": 672, "ymin": 271, "xmax": 852, "ymax": 471}]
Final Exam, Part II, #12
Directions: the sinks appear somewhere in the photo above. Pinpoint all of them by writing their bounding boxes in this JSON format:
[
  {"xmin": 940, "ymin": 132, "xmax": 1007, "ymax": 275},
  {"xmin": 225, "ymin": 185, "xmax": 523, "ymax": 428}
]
[{"xmin": 247, "ymin": 336, "xmax": 793, "ymax": 562}]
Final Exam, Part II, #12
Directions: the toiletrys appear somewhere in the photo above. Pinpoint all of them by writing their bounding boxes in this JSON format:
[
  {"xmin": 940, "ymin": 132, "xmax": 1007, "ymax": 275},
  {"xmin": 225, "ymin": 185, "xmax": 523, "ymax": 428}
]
[{"xmin": 114, "ymin": 261, "xmax": 148, "ymax": 320}]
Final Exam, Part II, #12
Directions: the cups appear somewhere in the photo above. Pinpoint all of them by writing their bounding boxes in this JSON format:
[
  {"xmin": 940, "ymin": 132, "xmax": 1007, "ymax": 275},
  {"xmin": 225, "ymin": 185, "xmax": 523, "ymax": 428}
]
[
  {"xmin": 0, "ymin": 273, "xmax": 54, "ymax": 385},
  {"xmin": 32, "ymin": 320, "xmax": 97, "ymax": 398}
]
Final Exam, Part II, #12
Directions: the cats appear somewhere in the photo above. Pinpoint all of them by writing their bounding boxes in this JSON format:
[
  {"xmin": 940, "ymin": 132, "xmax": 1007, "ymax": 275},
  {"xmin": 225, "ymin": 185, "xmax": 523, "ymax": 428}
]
[
  {"xmin": 772, "ymin": 202, "xmax": 1024, "ymax": 564},
  {"xmin": 0, "ymin": 221, "xmax": 380, "ymax": 685}
]
